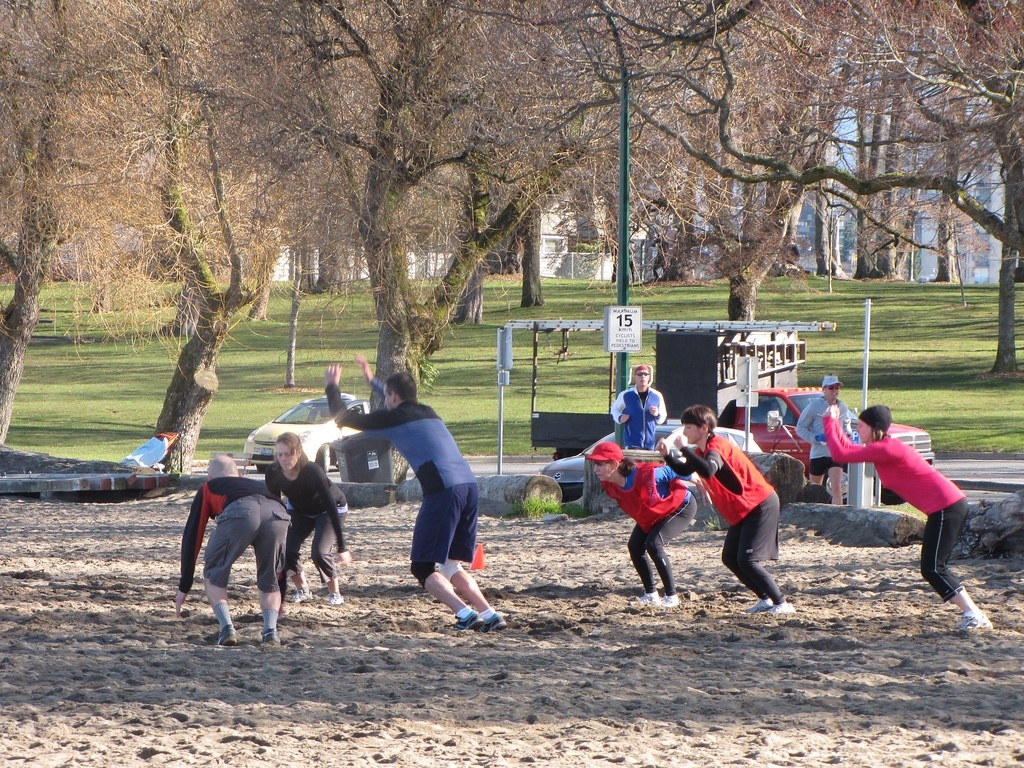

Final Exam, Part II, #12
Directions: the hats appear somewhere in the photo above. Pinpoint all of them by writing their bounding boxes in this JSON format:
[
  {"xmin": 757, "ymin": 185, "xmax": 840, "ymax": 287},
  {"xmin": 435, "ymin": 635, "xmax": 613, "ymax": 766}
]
[
  {"xmin": 822, "ymin": 375, "xmax": 841, "ymax": 391},
  {"xmin": 859, "ymin": 405, "xmax": 892, "ymax": 431},
  {"xmin": 585, "ymin": 441, "xmax": 623, "ymax": 460},
  {"xmin": 634, "ymin": 365, "xmax": 650, "ymax": 374}
]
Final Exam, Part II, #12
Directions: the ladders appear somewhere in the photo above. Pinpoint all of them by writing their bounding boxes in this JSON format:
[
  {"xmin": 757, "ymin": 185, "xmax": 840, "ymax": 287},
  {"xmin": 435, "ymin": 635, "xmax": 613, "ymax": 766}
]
[{"xmin": 720, "ymin": 338, "xmax": 807, "ymax": 387}]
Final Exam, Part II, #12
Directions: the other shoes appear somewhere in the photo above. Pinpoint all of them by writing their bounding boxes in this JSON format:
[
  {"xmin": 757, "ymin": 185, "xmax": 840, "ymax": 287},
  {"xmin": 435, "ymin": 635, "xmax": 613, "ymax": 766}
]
[
  {"xmin": 327, "ymin": 592, "xmax": 345, "ymax": 605},
  {"xmin": 662, "ymin": 594, "xmax": 679, "ymax": 608},
  {"xmin": 768, "ymin": 603, "xmax": 796, "ymax": 614},
  {"xmin": 260, "ymin": 627, "xmax": 282, "ymax": 643},
  {"xmin": 953, "ymin": 611, "xmax": 993, "ymax": 631},
  {"xmin": 628, "ymin": 591, "xmax": 661, "ymax": 607},
  {"xmin": 289, "ymin": 589, "xmax": 314, "ymax": 603},
  {"xmin": 744, "ymin": 600, "xmax": 774, "ymax": 614},
  {"xmin": 216, "ymin": 624, "xmax": 238, "ymax": 645}
]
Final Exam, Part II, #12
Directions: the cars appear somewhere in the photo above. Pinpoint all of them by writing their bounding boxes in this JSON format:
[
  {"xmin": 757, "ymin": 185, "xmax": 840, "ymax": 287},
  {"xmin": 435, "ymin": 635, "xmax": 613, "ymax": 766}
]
[
  {"xmin": 539, "ymin": 419, "xmax": 764, "ymax": 510},
  {"xmin": 243, "ymin": 391, "xmax": 371, "ymax": 473}
]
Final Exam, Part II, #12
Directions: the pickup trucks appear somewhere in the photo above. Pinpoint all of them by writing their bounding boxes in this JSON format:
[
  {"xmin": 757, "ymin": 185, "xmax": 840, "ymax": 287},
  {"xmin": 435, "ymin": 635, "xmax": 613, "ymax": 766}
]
[{"xmin": 529, "ymin": 384, "xmax": 935, "ymax": 507}]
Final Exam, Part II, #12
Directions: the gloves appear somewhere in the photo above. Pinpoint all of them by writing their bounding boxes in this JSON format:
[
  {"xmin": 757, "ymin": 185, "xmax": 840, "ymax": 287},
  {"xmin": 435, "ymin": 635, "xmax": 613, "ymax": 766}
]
[{"xmin": 816, "ymin": 433, "xmax": 826, "ymax": 442}]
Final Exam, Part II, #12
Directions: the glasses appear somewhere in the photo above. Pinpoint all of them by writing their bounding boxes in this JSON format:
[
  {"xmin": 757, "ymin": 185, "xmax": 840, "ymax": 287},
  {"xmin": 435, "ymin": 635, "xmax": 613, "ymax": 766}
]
[
  {"xmin": 590, "ymin": 460, "xmax": 608, "ymax": 466},
  {"xmin": 635, "ymin": 372, "xmax": 650, "ymax": 376},
  {"xmin": 826, "ymin": 386, "xmax": 839, "ymax": 390}
]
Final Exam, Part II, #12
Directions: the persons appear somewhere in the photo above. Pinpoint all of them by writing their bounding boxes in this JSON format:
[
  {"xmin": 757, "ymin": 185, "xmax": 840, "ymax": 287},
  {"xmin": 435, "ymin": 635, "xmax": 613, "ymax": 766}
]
[
  {"xmin": 177, "ymin": 456, "xmax": 292, "ymax": 645},
  {"xmin": 611, "ymin": 366, "xmax": 668, "ymax": 450},
  {"xmin": 656, "ymin": 405, "xmax": 796, "ymax": 614},
  {"xmin": 265, "ymin": 433, "xmax": 354, "ymax": 606},
  {"xmin": 585, "ymin": 441, "xmax": 697, "ymax": 609},
  {"xmin": 822, "ymin": 405, "xmax": 993, "ymax": 630},
  {"xmin": 796, "ymin": 375, "xmax": 854, "ymax": 505},
  {"xmin": 326, "ymin": 353, "xmax": 507, "ymax": 634}
]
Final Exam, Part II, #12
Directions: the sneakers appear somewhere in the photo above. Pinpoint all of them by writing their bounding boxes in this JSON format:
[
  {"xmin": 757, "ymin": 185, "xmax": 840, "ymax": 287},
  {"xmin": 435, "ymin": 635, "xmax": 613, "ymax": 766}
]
[
  {"xmin": 454, "ymin": 610, "xmax": 484, "ymax": 631},
  {"xmin": 478, "ymin": 613, "xmax": 508, "ymax": 632}
]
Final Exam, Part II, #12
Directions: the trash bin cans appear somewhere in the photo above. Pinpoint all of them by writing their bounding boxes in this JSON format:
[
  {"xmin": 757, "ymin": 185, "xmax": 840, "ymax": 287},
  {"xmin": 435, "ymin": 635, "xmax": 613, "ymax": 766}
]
[{"xmin": 329, "ymin": 432, "xmax": 395, "ymax": 482}]
